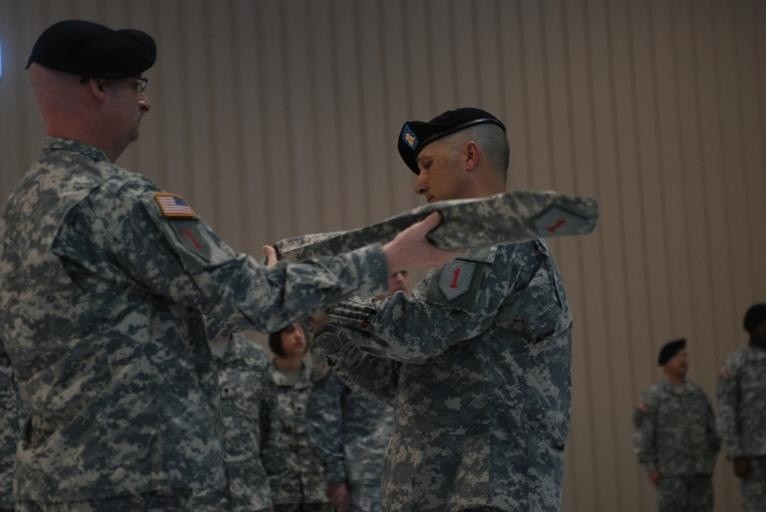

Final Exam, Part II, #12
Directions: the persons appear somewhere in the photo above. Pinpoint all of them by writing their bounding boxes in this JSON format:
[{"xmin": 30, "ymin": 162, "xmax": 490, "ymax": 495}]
[
  {"xmin": 631, "ymin": 336, "xmax": 723, "ymax": 511},
  {"xmin": 259, "ymin": 323, "xmax": 331, "ymax": 512},
  {"xmin": 308, "ymin": 367, "xmax": 401, "ymax": 510},
  {"xmin": 1, "ymin": 17, "xmax": 469, "ymax": 511},
  {"xmin": 717, "ymin": 301, "xmax": 766, "ymax": 512},
  {"xmin": 328, "ymin": 106, "xmax": 574, "ymax": 510},
  {"xmin": 207, "ymin": 327, "xmax": 279, "ymax": 512}
]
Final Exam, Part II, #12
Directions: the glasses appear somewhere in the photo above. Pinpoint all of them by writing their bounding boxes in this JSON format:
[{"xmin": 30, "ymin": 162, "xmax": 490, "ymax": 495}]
[{"xmin": 104, "ymin": 75, "xmax": 148, "ymax": 94}]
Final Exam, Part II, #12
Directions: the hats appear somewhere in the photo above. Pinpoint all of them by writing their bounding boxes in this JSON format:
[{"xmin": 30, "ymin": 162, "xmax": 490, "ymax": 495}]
[
  {"xmin": 398, "ymin": 108, "xmax": 506, "ymax": 176},
  {"xmin": 743, "ymin": 304, "xmax": 766, "ymax": 330},
  {"xmin": 25, "ymin": 20, "xmax": 156, "ymax": 81},
  {"xmin": 658, "ymin": 339, "xmax": 686, "ymax": 365}
]
